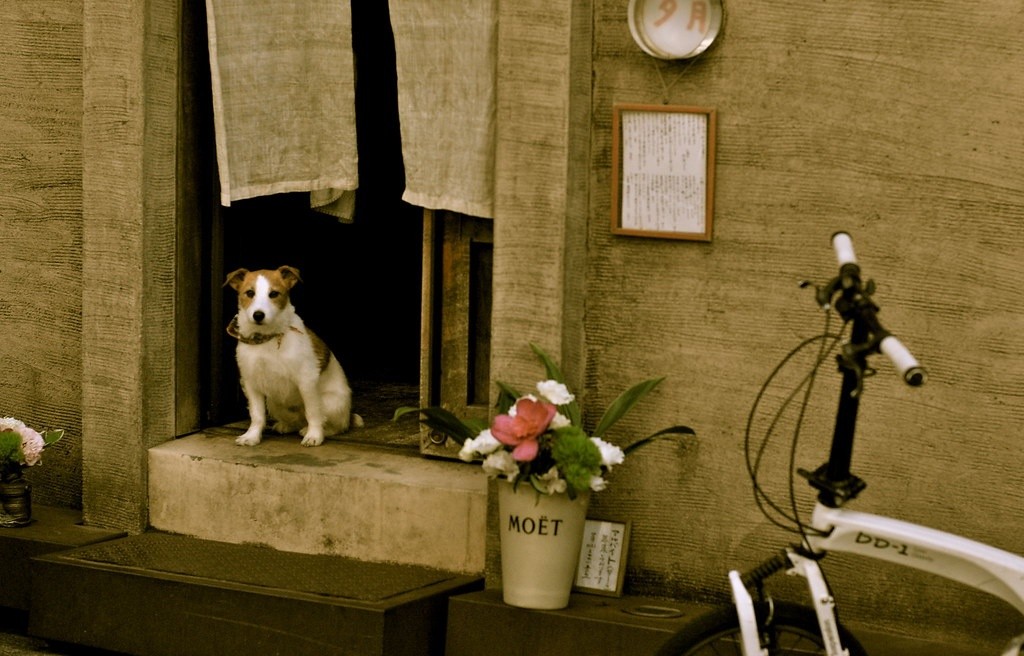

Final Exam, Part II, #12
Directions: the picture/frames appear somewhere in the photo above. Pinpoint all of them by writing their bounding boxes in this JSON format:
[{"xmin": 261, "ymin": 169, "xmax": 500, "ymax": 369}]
[
  {"xmin": 611, "ymin": 101, "xmax": 714, "ymax": 244},
  {"xmin": 571, "ymin": 514, "xmax": 630, "ymax": 597}
]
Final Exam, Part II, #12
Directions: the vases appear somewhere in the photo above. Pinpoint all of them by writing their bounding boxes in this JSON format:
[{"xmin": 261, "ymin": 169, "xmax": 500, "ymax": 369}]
[
  {"xmin": 0, "ymin": 469, "xmax": 32, "ymax": 527},
  {"xmin": 496, "ymin": 479, "xmax": 587, "ymax": 609}
]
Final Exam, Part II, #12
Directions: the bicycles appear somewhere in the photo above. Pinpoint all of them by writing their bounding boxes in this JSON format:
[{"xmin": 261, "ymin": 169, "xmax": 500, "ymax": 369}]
[{"xmin": 650, "ymin": 227, "xmax": 1024, "ymax": 656}]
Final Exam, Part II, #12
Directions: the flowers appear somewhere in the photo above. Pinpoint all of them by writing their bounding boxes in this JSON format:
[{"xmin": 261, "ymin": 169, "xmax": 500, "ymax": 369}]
[
  {"xmin": 0, "ymin": 415, "xmax": 65, "ymax": 469},
  {"xmin": 391, "ymin": 345, "xmax": 697, "ymax": 491}
]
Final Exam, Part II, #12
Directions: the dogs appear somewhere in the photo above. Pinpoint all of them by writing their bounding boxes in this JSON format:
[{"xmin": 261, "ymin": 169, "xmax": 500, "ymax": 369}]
[{"xmin": 222, "ymin": 266, "xmax": 365, "ymax": 447}]
